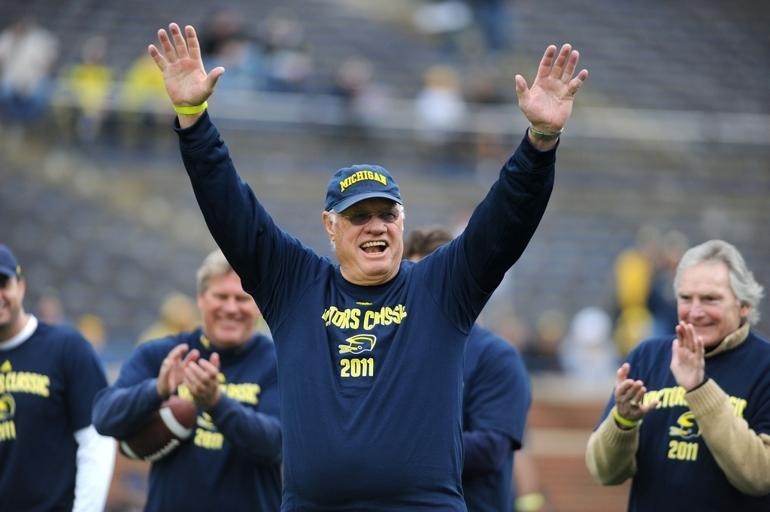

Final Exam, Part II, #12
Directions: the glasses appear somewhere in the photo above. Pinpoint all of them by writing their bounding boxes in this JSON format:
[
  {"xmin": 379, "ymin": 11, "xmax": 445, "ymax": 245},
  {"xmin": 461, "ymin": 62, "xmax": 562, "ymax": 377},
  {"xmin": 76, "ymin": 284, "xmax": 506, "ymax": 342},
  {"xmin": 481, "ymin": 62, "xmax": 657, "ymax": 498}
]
[{"xmin": 338, "ymin": 208, "xmax": 399, "ymax": 224}]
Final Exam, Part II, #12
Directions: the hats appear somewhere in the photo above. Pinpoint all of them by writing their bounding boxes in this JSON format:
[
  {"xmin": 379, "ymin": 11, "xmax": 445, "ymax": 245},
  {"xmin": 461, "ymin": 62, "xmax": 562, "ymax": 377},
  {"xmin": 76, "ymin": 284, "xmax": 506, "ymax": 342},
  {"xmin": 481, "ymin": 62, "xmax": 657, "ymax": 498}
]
[
  {"xmin": 324, "ymin": 163, "xmax": 402, "ymax": 214},
  {"xmin": 0, "ymin": 245, "xmax": 22, "ymax": 277}
]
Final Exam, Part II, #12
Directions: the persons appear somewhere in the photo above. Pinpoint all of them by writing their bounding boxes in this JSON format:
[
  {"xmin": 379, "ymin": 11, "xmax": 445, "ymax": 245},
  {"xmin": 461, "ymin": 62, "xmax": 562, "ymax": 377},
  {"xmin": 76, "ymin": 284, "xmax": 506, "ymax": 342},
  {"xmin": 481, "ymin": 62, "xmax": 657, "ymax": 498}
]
[
  {"xmin": 405, "ymin": 226, "xmax": 530, "ymax": 511},
  {"xmin": 583, "ymin": 240, "xmax": 770, "ymax": 511},
  {"xmin": 149, "ymin": 22, "xmax": 589, "ymax": 512},
  {"xmin": 0, "ymin": 243, "xmax": 118, "ymax": 512},
  {"xmin": 92, "ymin": 248, "xmax": 282, "ymax": 512}
]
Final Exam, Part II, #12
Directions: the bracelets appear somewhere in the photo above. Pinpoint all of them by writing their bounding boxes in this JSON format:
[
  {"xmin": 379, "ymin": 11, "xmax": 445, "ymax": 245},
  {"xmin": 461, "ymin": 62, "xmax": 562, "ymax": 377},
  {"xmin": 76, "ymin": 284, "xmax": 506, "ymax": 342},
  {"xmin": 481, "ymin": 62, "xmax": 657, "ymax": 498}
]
[
  {"xmin": 172, "ymin": 103, "xmax": 211, "ymax": 115},
  {"xmin": 613, "ymin": 407, "xmax": 643, "ymax": 430}
]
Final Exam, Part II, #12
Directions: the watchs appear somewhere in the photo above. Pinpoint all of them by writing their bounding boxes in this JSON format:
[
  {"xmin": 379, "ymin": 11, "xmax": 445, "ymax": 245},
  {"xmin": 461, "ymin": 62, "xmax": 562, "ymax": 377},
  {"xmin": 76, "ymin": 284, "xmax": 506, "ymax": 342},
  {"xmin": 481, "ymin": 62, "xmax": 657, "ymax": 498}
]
[{"xmin": 529, "ymin": 126, "xmax": 564, "ymax": 140}]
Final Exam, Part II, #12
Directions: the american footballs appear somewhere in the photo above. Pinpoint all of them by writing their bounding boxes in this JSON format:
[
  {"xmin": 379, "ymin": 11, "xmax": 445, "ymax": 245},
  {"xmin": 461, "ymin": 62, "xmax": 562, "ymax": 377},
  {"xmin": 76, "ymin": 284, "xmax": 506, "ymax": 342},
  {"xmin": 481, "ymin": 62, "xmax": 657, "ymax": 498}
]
[{"xmin": 117, "ymin": 395, "xmax": 198, "ymax": 463}]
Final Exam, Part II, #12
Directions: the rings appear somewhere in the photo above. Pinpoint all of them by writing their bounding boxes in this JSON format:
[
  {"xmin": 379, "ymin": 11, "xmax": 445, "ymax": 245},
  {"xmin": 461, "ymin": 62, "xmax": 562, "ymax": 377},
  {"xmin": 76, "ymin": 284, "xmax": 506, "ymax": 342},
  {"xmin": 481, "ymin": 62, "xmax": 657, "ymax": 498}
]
[{"xmin": 629, "ymin": 399, "xmax": 638, "ymax": 408}]
